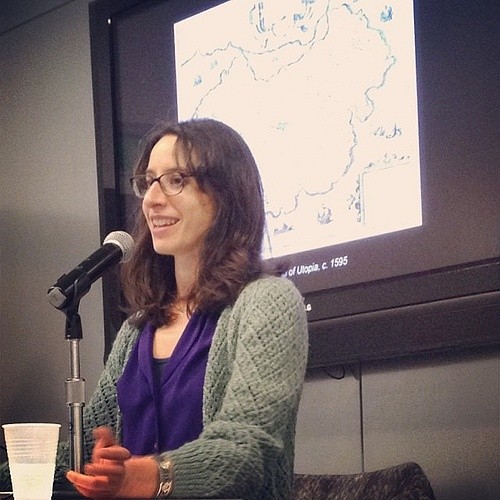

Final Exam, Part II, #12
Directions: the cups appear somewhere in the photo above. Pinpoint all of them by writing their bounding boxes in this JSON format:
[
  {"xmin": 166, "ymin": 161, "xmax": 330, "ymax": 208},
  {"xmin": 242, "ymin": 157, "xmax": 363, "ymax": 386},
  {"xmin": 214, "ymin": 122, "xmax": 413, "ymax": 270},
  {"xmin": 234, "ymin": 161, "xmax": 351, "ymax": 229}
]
[{"xmin": 2, "ymin": 423, "xmax": 61, "ymax": 500}]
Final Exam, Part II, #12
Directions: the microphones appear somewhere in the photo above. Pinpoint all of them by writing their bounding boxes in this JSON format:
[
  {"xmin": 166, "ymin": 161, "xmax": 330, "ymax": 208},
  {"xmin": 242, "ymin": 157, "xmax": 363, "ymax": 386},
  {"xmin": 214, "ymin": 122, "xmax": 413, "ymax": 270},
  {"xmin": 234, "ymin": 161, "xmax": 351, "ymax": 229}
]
[{"xmin": 47, "ymin": 231, "xmax": 135, "ymax": 309}]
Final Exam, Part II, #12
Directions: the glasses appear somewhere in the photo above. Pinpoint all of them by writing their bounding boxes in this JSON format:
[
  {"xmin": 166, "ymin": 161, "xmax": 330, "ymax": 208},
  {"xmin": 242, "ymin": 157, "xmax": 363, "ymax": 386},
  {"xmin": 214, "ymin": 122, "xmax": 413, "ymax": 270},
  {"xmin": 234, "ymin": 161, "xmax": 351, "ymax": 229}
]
[{"xmin": 129, "ymin": 172, "xmax": 217, "ymax": 197}]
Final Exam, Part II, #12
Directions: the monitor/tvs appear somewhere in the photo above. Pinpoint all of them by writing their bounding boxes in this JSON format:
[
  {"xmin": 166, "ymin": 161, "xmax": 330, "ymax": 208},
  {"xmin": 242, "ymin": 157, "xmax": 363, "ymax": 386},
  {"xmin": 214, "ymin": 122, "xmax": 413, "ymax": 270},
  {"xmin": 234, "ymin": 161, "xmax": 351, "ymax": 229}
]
[{"xmin": 85, "ymin": 0, "xmax": 500, "ymax": 370}]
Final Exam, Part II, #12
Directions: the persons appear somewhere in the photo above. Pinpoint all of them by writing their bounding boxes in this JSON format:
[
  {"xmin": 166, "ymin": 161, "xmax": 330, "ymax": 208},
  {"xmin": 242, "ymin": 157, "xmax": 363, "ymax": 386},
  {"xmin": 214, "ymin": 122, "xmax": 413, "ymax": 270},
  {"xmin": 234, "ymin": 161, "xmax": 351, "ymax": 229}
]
[{"xmin": 1, "ymin": 119, "xmax": 310, "ymax": 499}]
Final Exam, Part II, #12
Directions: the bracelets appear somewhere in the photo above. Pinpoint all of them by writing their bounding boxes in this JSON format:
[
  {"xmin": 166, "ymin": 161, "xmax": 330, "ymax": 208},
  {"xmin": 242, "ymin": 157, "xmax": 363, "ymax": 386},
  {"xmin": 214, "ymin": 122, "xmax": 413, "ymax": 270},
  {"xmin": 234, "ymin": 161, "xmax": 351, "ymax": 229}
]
[{"xmin": 149, "ymin": 453, "xmax": 174, "ymax": 498}]
